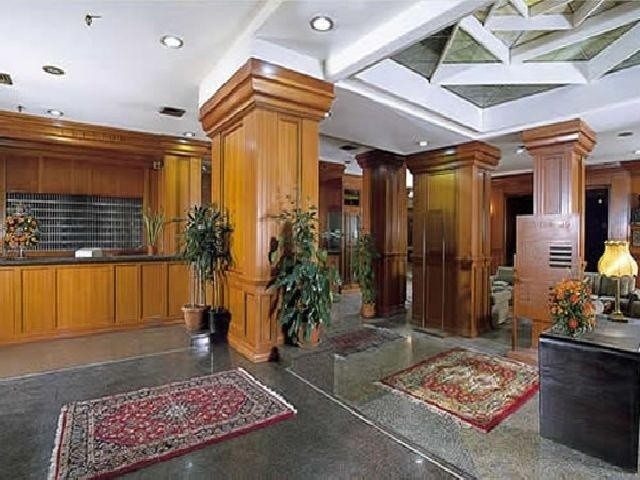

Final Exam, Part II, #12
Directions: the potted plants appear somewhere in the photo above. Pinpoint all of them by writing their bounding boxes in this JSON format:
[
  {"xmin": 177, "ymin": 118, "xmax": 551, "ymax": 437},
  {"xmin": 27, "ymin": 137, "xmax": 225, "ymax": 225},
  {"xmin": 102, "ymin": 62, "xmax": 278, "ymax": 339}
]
[
  {"xmin": 142, "ymin": 205, "xmax": 168, "ymax": 255},
  {"xmin": 351, "ymin": 233, "xmax": 379, "ymax": 319},
  {"xmin": 263, "ymin": 191, "xmax": 343, "ymax": 350},
  {"xmin": 171, "ymin": 203, "xmax": 233, "ymax": 343}
]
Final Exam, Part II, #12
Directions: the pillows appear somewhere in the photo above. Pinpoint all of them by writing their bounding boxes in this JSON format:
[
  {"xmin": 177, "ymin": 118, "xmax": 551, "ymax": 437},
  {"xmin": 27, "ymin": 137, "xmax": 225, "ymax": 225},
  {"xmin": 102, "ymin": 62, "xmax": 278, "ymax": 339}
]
[{"xmin": 590, "ymin": 273, "xmax": 629, "ymax": 297}]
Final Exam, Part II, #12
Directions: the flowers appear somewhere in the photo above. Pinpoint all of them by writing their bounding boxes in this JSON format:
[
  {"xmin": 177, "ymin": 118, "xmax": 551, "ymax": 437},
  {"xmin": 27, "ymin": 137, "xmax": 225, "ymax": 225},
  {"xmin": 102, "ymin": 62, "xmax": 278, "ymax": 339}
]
[
  {"xmin": 0, "ymin": 201, "xmax": 43, "ymax": 254},
  {"xmin": 544, "ymin": 258, "xmax": 598, "ymax": 336}
]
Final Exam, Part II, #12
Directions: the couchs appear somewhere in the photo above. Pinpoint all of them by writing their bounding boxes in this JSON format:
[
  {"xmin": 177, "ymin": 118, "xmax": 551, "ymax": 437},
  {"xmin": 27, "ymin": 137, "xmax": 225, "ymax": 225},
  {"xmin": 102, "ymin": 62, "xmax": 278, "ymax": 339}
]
[{"xmin": 585, "ymin": 272, "xmax": 639, "ymax": 318}]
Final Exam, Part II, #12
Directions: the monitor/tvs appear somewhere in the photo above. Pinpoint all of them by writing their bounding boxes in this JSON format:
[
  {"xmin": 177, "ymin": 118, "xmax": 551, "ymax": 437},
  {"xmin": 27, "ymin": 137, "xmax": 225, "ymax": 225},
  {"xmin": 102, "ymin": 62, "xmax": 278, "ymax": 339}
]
[{"xmin": 75, "ymin": 247, "xmax": 102, "ymax": 258}]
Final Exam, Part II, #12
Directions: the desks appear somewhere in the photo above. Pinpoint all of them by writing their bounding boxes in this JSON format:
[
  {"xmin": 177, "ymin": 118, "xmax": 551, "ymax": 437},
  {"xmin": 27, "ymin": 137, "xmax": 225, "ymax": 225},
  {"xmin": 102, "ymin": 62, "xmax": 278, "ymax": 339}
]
[{"xmin": 538, "ymin": 315, "xmax": 640, "ymax": 472}]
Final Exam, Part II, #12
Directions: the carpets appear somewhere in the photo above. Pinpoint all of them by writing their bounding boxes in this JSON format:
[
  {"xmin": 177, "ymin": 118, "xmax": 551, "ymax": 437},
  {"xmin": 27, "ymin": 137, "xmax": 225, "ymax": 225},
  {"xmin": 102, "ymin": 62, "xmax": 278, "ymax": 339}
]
[
  {"xmin": 372, "ymin": 345, "xmax": 538, "ymax": 434},
  {"xmin": 323, "ymin": 324, "xmax": 403, "ymax": 360},
  {"xmin": 47, "ymin": 365, "xmax": 299, "ymax": 480}
]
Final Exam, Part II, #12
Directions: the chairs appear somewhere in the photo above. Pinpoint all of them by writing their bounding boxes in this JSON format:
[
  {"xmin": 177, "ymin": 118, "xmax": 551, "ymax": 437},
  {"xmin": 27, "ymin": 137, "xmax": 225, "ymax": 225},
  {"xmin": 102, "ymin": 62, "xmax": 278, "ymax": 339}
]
[{"xmin": 489, "ymin": 265, "xmax": 514, "ymax": 305}]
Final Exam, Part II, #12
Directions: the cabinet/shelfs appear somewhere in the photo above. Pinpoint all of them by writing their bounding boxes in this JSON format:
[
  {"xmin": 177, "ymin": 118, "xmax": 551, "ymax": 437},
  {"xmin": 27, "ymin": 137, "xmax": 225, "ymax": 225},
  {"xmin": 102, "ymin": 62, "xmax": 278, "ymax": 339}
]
[
  {"xmin": 0, "ymin": 267, "xmax": 58, "ymax": 342},
  {"xmin": 57, "ymin": 265, "xmax": 116, "ymax": 331},
  {"xmin": 115, "ymin": 262, "xmax": 191, "ymax": 325}
]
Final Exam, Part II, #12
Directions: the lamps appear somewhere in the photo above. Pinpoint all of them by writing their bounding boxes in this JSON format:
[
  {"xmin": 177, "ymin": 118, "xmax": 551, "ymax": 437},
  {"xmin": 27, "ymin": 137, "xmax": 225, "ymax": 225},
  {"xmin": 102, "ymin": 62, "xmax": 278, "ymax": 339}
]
[{"xmin": 596, "ymin": 238, "xmax": 640, "ymax": 325}]
